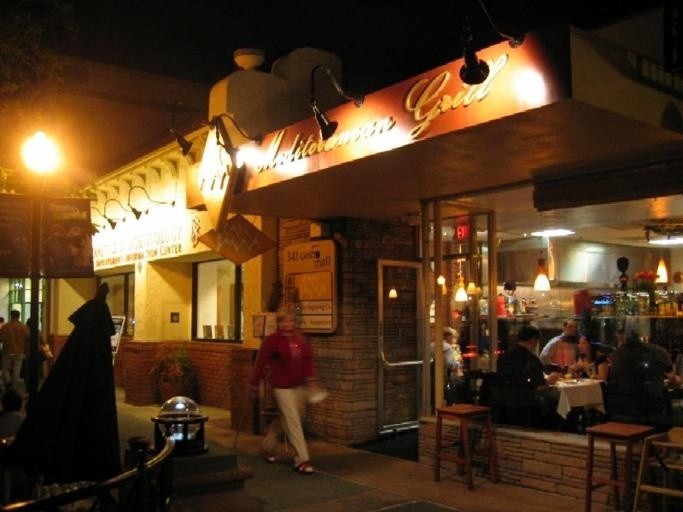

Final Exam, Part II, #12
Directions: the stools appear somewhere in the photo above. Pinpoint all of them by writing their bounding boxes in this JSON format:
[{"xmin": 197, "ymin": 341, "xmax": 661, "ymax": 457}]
[
  {"xmin": 585, "ymin": 422, "xmax": 654, "ymax": 512},
  {"xmin": 433, "ymin": 403, "xmax": 496, "ymax": 490}
]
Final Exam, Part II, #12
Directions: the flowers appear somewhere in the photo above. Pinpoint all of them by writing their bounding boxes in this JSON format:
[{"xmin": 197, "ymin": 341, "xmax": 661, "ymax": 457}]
[{"xmin": 633, "ymin": 271, "xmax": 659, "ymax": 295}]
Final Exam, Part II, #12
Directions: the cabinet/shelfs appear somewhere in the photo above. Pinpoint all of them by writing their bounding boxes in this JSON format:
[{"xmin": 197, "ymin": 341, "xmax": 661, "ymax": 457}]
[{"xmin": 568, "ymin": 314, "xmax": 683, "ymax": 355}]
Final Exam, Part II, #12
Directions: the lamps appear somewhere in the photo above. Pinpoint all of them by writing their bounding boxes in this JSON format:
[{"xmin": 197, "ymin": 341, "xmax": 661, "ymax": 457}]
[
  {"xmin": 127, "ymin": 186, "xmax": 175, "ymax": 220},
  {"xmin": 459, "ymin": 2, "xmax": 524, "ymax": 85},
  {"xmin": 455, "ymin": 244, "xmax": 467, "ymax": 302},
  {"xmin": 168, "ymin": 104, "xmax": 201, "ymax": 155},
  {"xmin": 209, "ymin": 114, "xmax": 262, "ymax": 169},
  {"xmin": 533, "ymin": 236, "xmax": 552, "ymax": 292},
  {"xmin": 653, "ymin": 248, "xmax": 670, "ymax": 284},
  {"xmin": 89, "ymin": 206, "xmax": 117, "ymax": 229},
  {"xmin": 104, "ymin": 198, "xmax": 149, "ymax": 229},
  {"xmin": 306, "ymin": 64, "xmax": 364, "ymax": 140}
]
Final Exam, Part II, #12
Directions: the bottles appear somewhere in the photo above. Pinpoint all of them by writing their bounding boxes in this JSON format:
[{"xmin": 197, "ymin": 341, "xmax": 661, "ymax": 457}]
[
  {"xmin": 497, "ymin": 292, "xmax": 505, "ymax": 314},
  {"xmin": 612, "ymin": 294, "xmax": 651, "ymax": 318},
  {"xmin": 655, "ymin": 290, "xmax": 683, "ymax": 317},
  {"xmin": 521, "ymin": 297, "xmax": 525, "ymax": 314},
  {"xmin": 481, "ymin": 351, "xmax": 490, "ymax": 369}
]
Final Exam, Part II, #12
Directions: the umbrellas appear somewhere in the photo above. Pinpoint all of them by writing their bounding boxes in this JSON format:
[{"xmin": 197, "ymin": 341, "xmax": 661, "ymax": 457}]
[{"xmin": 10, "ymin": 282, "xmax": 121, "ymax": 480}]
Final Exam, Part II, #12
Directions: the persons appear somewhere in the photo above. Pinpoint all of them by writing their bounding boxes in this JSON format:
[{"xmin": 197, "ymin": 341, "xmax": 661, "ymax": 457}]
[
  {"xmin": 495, "ymin": 325, "xmax": 557, "ymax": 394},
  {"xmin": 1, "ymin": 310, "xmax": 52, "ymax": 396},
  {"xmin": 667, "ymin": 352, "xmax": 683, "ymax": 389},
  {"xmin": 606, "ymin": 319, "xmax": 674, "ymax": 425},
  {"xmin": 431, "ymin": 326, "xmax": 459, "ymax": 372},
  {"xmin": 539, "ymin": 318, "xmax": 578, "ymax": 368},
  {"xmin": 250, "ymin": 305, "xmax": 320, "ymax": 474},
  {"xmin": 568, "ymin": 334, "xmax": 609, "ymax": 380},
  {"xmin": 503, "ymin": 282, "xmax": 515, "ymax": 298}
]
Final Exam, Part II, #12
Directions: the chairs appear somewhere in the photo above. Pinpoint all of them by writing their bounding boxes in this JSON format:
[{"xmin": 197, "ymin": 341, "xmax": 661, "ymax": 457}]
[
  {"xmin": 632, "ymin": 426, "xmax": 683, "ymax": 512},
  {"xmin": 480, "ymin": 368, "xmax": 542, "ymax": 427}
]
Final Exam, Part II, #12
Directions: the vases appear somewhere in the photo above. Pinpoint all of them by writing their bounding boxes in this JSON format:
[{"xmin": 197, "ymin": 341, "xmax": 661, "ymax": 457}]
[{"xmin": 648, "ymin": 291, "xmax": 659, "ymax": 315}]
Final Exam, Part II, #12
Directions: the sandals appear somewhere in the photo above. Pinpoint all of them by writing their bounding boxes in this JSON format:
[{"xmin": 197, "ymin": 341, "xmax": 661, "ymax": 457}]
[{"xmin": 294, "ymin": 461, "xmax": 314, "ymax": 474}]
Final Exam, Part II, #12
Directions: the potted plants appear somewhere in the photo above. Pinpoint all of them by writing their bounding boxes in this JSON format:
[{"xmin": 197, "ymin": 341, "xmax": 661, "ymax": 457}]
[{"xmin": 149, "ymin": 342, "xmax": 197, "ymax": 405}]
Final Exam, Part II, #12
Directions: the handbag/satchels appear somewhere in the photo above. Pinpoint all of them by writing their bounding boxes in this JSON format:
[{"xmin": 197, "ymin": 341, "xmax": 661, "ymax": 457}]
[{"xmin": 42, "ymin": 344, "xmax": 53, "ymax": 358}]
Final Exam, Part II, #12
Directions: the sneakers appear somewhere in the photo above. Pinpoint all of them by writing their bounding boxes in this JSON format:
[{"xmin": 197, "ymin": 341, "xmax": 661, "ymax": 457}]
[{"xmin": 261, "ymin": 449, "xmax": 277, "ymax": 462}]
